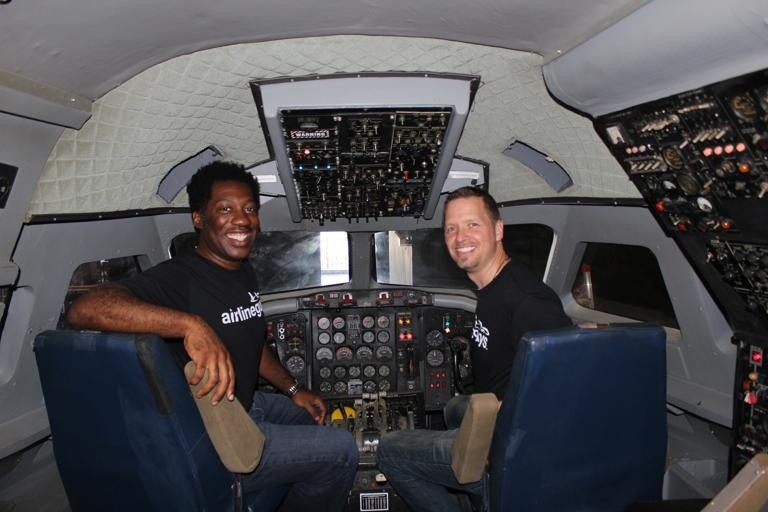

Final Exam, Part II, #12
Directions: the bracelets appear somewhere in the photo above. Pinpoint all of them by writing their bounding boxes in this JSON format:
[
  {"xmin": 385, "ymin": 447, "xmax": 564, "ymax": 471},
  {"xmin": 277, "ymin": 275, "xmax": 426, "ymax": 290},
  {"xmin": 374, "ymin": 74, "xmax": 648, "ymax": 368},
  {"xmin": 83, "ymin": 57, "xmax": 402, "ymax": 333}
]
[{"xmin": 283, "ymin": 378, "xmax": 306, "ymax": 398}]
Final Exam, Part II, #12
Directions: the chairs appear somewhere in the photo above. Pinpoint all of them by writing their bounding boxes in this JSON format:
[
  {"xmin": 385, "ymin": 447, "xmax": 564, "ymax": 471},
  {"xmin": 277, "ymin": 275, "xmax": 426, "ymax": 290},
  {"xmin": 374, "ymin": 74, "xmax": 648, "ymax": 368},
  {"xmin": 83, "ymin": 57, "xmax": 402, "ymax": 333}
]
[
  {"xmin": 454, "ymin": 324, "xmax": 667, "ymax": 511},
  {"xmin": 30, "ymin": 326, "xmax": 268, "ymax": 512}
]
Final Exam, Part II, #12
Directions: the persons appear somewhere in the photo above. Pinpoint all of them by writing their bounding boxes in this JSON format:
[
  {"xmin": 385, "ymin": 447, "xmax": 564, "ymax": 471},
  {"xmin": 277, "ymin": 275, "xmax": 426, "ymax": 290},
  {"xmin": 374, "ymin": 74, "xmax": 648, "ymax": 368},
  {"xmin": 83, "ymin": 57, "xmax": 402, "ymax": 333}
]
[
  {"xmin": 67, "ymin": 161, "xmax": 360, "ymax": 512},
  {"xmin": 375, "ymin": 185, "xmax": 573, "ymax": 511}
]
